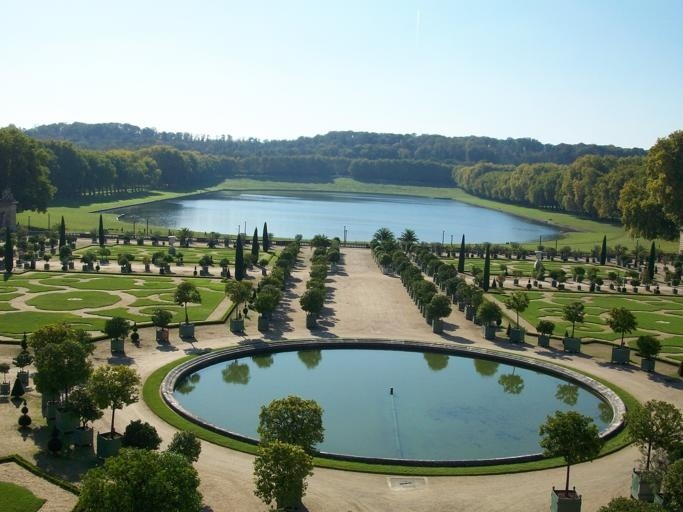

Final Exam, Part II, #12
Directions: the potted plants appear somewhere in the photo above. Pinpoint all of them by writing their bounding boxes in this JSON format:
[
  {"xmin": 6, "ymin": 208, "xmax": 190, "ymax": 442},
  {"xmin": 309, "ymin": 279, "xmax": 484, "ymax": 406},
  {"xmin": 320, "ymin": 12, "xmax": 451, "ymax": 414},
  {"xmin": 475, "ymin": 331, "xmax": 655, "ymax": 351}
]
[
  {"xmin": 537, "ymin": 401, "xmax": 681, "ymax": 511},
  {"xmin": 371, "ymin": 228, "xmax": 683, "ymax": 295},
  {"xmin": 101, "ymin": 279, "xmax": 202, "ymax": 355},
  {"xmin": 220, "ymin": 236, "xmax": 339, "ymax": 335},
  {"xmin": 0, "ymin": 324, "xmax": 320, "ymax": 511},
  {"xmin": 606, "ymin": 307, "xmax": 661, "ymax": 370},
  {"xmin": 411, "ymin": 289, "xmax": 585, "ymax": 352},
  {"xmin": 1, "ymin": 227, "xmax": 220, "ymax": 279}
]
